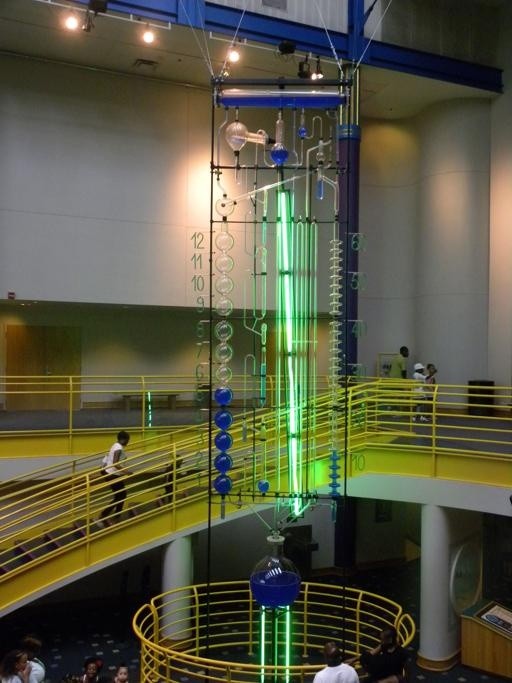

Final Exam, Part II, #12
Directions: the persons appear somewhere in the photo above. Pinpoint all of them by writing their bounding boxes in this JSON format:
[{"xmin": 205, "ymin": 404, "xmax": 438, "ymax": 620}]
[
  {"xmin": 423, "ymin": 362, "xmax": 438, "ymax": 402},
  {"xmin": 357, "ymin": 626, "xmax": 404, "ymax": 682},
  {"xmin": 21, "ymin": 635, "xmax": 49, "ymax": 683},
  {"xmin": 382, "ymin": 344, "xmax": 408, "ymax": 421},
  {"xmin": 408, "ymin": 362, "xmax": 433, "ymax": 424},
  {"xmin": 65, "ymin": 655, "xmax": 132, "ymax": 683},
  {"xmin": 310, "ymin": 640, "xmax": 360, "ymax": 683},
  {"xmin": 94, "ymin": 430, "xmax": 135, "ymax": 529},
  {"xmin": 154, "ymin": 451, "xmax": 184, "ymax": 505},
  {"xmin": 0, "ymin": 647, "xmax": 28, "ymax": 683}
]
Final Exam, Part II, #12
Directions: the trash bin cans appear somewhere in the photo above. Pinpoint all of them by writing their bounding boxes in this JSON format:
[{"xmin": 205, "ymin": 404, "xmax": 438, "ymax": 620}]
[
  {"xmin": 196, "ymin": 382, "xmax": 214, "ymax": 409},
  {"xmin": 468, "ymin": 380, "xmax": 495, "ymax": 417}
]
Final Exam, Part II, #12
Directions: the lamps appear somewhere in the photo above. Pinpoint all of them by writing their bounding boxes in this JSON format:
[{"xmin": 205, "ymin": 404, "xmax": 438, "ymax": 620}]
[
  {"xmin": 81, "ymin": 9, "xmax": 95, "ymax": 32},
  {"xmin": 297, "ymin": 54, "xmax": 324, "ymax": 80}
]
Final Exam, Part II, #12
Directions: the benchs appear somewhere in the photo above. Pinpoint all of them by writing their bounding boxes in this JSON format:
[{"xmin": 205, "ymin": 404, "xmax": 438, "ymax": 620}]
[{"xmin": 122, "ymin": 393, "xmax": 180, "ymax": 411}]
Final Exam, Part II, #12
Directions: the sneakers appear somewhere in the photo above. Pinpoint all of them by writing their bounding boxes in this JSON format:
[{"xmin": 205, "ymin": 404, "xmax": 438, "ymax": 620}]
[{"xmin": 411, "ymin": 415, "xmax": 433, "ymax": 422}]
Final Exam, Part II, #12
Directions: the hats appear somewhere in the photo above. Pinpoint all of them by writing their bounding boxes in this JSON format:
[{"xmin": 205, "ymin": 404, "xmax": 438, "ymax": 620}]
[{"xmin": 413, "ymin": 362, "xmax": 425, "ymax": 370}]
